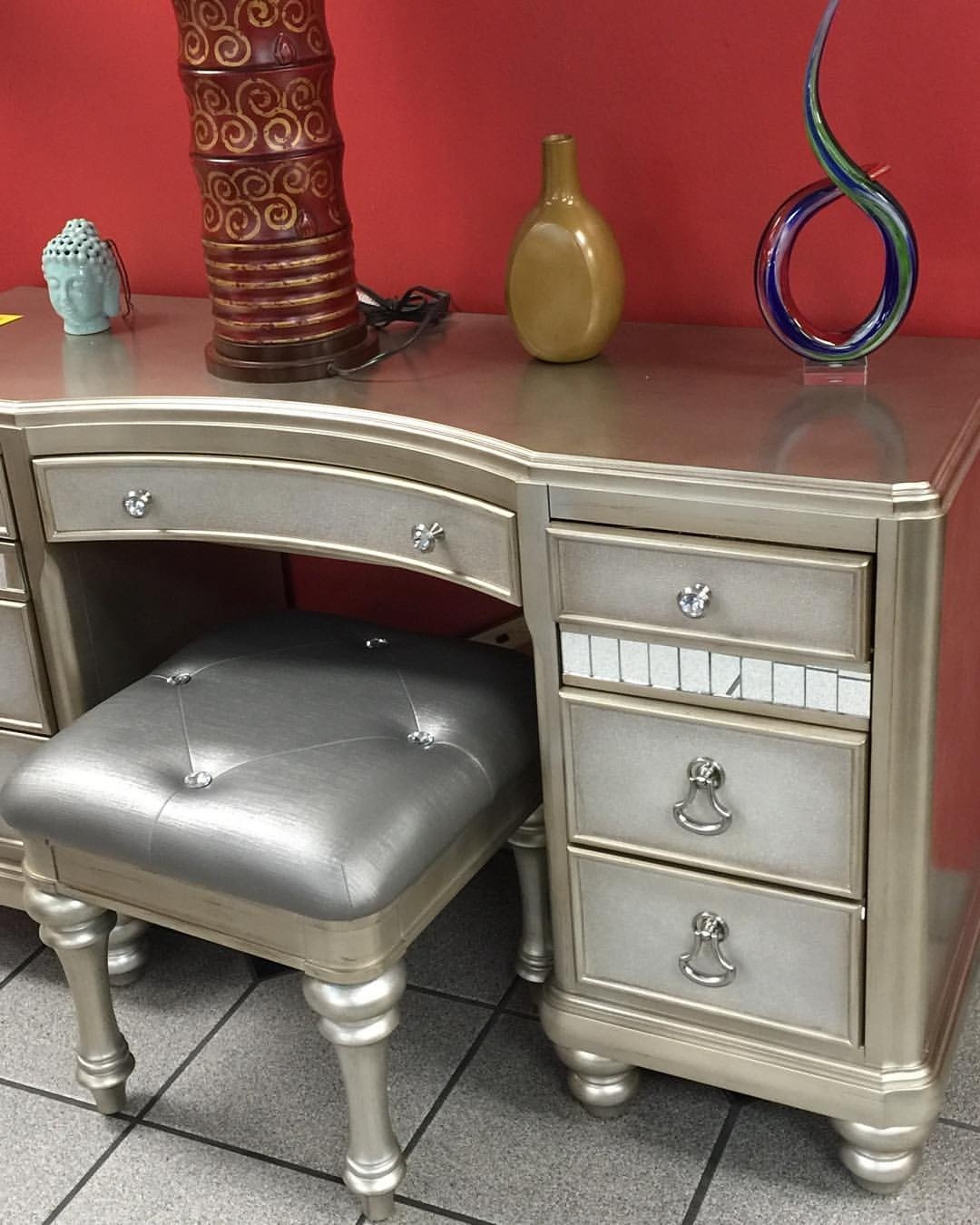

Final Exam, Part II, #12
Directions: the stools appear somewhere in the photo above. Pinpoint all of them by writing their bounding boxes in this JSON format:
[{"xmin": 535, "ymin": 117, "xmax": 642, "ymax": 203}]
[{"xmin": 1, "ymin": 609, "xmax": 554, "ymax": 1220}]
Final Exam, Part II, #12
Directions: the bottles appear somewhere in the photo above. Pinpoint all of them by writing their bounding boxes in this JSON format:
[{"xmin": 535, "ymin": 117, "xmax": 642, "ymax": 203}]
[{"xmin": 505, "ymin": 134, "xmax": 625, "ymax": 363}]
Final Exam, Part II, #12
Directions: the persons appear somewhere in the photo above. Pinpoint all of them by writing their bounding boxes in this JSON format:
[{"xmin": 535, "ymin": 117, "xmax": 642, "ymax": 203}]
[{"xmin": 40, "ymin": 217, "xmax": 123, "ymax": 335}]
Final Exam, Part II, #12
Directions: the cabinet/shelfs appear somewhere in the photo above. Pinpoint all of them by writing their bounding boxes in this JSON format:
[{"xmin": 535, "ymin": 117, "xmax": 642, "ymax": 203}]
[{"xmin": 0, "ymin": 281, "xmax": 980, "ymax": 1195}]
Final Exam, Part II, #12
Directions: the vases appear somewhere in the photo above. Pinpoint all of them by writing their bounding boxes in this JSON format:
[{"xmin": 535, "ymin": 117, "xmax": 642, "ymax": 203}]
[{"xmin": 503, "ymin": 133, "xmax": 625, "ymax": 363}]
